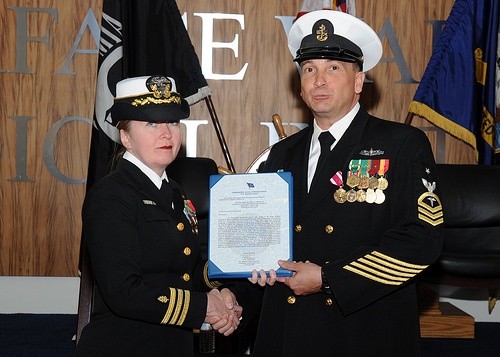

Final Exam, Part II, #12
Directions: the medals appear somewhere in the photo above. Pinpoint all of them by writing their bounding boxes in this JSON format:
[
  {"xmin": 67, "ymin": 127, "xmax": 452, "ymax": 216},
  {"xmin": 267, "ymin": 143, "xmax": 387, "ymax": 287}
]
[
  {"xmin": 183, "ymin": 199, "xmax": 200, "ymax": 234},
  {"xmin": 325, "ymin": 158, "xmax": 390, "ymax": 204}
]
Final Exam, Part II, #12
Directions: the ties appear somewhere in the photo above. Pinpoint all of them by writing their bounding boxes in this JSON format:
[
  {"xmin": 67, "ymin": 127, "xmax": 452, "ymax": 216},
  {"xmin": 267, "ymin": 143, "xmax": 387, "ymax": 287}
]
[
  {"xmin": 161, "ymin": 179, "xmax": 176, "ymax": 202},
  {"xmin": 316, "ymin": 131, "xmax": 336, "ymax": 168}
]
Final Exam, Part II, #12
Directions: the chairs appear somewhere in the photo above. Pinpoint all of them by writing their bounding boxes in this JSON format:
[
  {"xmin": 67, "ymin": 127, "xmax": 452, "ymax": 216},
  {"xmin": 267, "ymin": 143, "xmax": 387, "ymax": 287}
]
[{"xmin": 420, "ymin": 163, "xmax": 500, "ymax": 300}]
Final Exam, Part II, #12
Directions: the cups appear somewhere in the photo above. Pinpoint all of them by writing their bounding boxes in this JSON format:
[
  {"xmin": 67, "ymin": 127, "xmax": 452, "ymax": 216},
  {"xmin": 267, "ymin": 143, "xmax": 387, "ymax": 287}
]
[{"xmin": 199, "ymin": 322, "xmax": 214, "ymax": 354}]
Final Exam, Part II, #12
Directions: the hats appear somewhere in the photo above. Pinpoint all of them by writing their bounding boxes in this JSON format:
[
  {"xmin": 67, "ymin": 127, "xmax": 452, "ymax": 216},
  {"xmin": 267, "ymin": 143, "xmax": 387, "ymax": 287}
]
[
  {"xmin": 112, "ymin": 76, "xmax": 189, "ymax": 123},
  {"xmin": 288, "ymin": 10, "xmax": 383, "ymax": 73}
]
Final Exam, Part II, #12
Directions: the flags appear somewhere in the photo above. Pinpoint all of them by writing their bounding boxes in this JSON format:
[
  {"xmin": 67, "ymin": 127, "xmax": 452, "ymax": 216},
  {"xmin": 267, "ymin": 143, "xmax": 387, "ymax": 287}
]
[
  {"xmin": 409, "ymin": 0, "xmax": 500, "ymax": 163},
  {"xmin": 77, "ymin": 0, "xmax": 213, "ymax": 279}
]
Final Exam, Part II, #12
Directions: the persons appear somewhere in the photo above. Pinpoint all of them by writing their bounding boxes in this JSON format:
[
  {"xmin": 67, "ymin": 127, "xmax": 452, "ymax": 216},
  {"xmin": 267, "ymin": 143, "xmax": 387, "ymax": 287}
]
[
  {"xmin": 204, "ymin": 10, "xmax": 447, "ymax": 357},
  {"xmin": 77, "ymin": 46, "xmax": 241, "ymax": 357}
]
